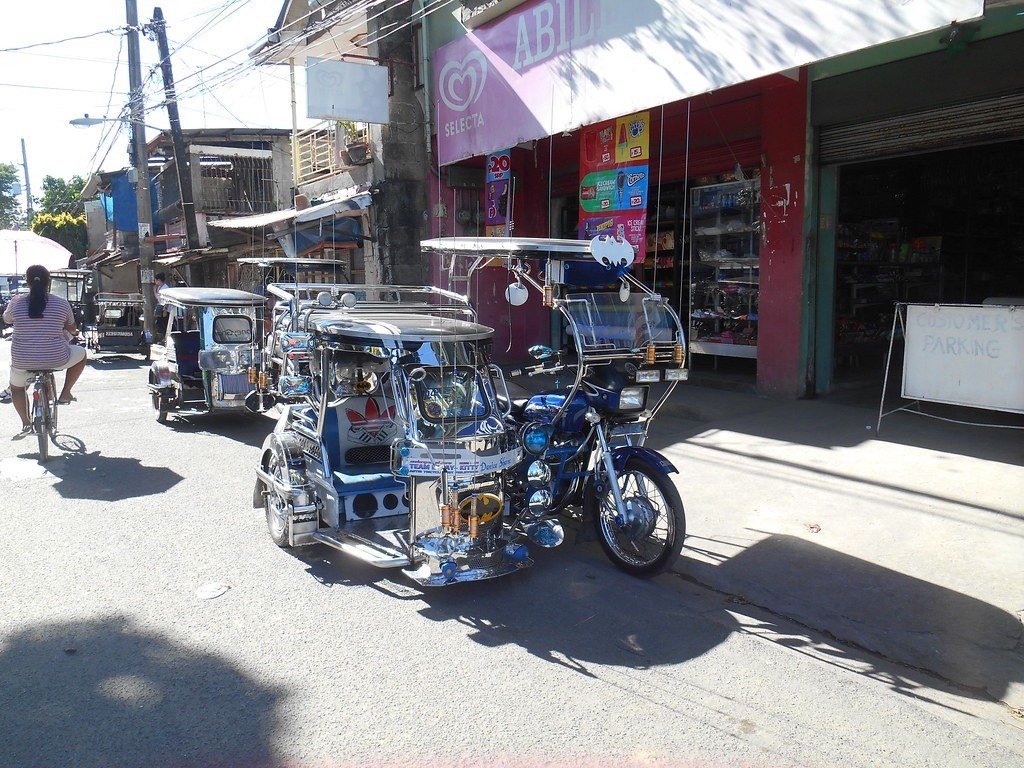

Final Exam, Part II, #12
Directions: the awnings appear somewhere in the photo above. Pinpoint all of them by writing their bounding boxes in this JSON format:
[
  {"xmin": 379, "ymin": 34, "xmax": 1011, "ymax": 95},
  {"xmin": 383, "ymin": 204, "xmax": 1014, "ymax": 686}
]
[{"xmin": 207, "ymin": 191, "xmax": 372, "ymax": 228}]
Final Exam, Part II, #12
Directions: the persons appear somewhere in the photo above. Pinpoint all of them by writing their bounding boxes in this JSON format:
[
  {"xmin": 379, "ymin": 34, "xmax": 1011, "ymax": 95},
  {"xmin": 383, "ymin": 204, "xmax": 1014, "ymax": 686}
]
[
  {"xmin": 153, "ymin": 272, "xmax": 171, "ymax": 337},
  {"xmin": 3, "ymin": 265, "xmax": 87, "ymax": 432},
  {"xmin": 116, "ymin": 306, "xmax": 139, "ymax": 328}
]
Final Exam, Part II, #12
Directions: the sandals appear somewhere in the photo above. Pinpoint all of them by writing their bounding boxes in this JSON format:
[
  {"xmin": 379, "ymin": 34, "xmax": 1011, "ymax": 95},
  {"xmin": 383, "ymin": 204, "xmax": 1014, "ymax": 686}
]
[{"xmin": 0, "ymin": 387, "xmax": 13, "ymax": 404}]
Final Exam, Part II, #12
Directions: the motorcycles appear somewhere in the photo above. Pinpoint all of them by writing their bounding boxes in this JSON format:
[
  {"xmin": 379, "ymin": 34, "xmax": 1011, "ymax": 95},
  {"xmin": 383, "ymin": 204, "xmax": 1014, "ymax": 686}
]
[{"xmin": 90, "ymin": 236, "xmax": 690, "ymax": 588}]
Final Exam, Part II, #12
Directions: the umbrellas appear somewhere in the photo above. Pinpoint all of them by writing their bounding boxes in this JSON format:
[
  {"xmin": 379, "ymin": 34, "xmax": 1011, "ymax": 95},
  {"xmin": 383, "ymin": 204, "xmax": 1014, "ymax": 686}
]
[{"xmin": 1, "ymin": 229, "xmax": 73, "ymax": 295}]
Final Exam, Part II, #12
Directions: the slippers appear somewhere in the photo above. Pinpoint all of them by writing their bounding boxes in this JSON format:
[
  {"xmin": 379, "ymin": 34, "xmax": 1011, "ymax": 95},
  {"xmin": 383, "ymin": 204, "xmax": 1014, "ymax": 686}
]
[
  {"xmin": 59, "ymin": 394, "xmax": 78, "ymax": 405},
  {"xmin": 10, "ymin": 424, "xmax": 32, "ymax": 440}
]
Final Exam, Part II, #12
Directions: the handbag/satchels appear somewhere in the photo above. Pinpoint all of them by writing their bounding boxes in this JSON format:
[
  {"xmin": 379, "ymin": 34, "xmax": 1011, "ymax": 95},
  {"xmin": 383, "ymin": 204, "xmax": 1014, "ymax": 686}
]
[{"xmin": 155, "ymin": 305, "xmax": 164, "ymax": 318}]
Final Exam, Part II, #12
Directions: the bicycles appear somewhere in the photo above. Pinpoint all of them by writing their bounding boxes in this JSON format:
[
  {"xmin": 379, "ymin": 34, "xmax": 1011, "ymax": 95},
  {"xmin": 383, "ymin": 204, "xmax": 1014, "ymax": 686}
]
[{"xmin": 25, "ymin": 365, "xmax": 60, "ymax": 468}]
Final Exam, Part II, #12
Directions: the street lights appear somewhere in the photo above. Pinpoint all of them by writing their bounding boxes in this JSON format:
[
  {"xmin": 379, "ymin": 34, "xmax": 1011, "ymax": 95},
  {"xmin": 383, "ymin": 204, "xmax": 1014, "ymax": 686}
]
[{"xmin": 71, "ymin": 116, "xmax": 193, "ymax": 291}]
